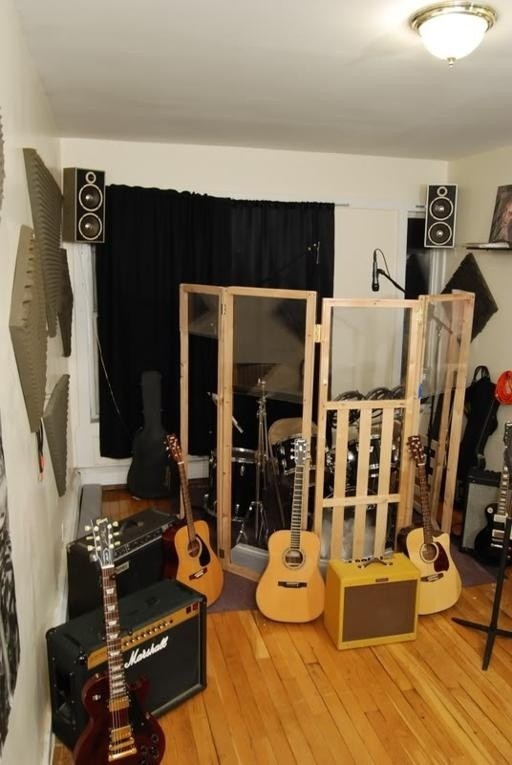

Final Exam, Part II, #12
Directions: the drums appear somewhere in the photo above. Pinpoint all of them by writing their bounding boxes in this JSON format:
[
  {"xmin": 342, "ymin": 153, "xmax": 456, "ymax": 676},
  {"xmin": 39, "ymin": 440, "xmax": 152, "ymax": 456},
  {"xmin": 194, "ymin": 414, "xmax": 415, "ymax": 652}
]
[
  {"xmin": 346, "ymin": 435, "xmax": 396, "ymax": 479},
  {"xmin": 324, "ymin": 446, "xmax": 376, "ymax": 513},
  {"xmin": 275, "ymin": 432, "xmax": 329, "ymax": 488},
  {"xmin": 204, "ymin": 448, "xmax": 267, "ymax": 522}
]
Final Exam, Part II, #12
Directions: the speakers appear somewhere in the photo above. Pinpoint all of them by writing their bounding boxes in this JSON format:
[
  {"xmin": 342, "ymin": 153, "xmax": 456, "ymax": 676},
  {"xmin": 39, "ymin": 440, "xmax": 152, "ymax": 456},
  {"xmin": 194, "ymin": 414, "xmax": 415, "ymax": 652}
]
[
  {"xmin": 62, "ymin": 168, "xmax": 106, "ymax": 244},
  {"xmin": 66, "ymin": 508, "xmax": 179, "ymax": 622},
  {"xmin": 460, "ymin": 470, "xmax": 512, "ymax": 553},
  {"xmin": 423, "ymin": 184, "xmax": 459, "ymax": 249},
  {"xmin": 45, "ymin": 581, "xmax": 208, "ymax": 756}
]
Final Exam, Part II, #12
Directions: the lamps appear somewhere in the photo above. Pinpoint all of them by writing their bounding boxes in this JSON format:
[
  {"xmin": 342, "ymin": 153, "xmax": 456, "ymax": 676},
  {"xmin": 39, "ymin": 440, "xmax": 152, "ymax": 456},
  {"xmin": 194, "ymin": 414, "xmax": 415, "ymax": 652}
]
[{"xmin": 409, "ymin": 0, "xmax": 499, "ymax": 66}]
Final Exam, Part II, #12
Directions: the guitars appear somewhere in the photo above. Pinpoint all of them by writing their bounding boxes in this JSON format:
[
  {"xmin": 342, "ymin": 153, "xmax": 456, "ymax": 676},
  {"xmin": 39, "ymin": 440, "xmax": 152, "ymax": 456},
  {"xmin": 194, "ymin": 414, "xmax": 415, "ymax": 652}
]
[
  {"xmin": 397, "ymin": 436, "xmax": 462, "ymax": 616},
  {"xmin": 474, "ymin": 420, "xmax": 512, "ymax": 567},
  {"xmin": 255, "ymin": 438, "xmax": 325, "ymax": 623},
  {"xmin": 74, "ymin": 515, "xmax": 165, "ymax": 764},
  {"xmin": 163, "ymin": 434, "xmax": 224, "ymax": 607}
]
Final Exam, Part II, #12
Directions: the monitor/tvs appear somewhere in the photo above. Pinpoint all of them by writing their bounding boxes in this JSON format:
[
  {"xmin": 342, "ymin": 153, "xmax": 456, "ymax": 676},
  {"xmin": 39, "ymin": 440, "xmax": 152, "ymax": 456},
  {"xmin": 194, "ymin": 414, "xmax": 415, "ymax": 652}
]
[{"xmin": 324, "ymin": 552, "xmax": 421, "ymax": 650}]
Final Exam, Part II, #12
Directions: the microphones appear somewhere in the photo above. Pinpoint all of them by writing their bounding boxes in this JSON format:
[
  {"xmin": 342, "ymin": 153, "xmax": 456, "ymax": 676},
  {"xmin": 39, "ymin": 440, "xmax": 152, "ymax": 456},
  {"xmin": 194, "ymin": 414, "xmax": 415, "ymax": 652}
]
[
  {"xmin": 315, "ymin": 241, "xmax": 320, "ymax": 265},
  {"xmin": 372, "ymin": 250, "xmax": 380, "ymax": 292}
]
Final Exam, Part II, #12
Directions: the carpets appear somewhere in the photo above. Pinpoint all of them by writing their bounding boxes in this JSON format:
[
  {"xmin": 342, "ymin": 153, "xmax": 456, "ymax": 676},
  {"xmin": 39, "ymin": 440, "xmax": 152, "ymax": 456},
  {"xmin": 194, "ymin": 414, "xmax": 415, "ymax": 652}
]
[{"xmin": 205, "ymin": 495, "xmax": 508, "ymax": 613}]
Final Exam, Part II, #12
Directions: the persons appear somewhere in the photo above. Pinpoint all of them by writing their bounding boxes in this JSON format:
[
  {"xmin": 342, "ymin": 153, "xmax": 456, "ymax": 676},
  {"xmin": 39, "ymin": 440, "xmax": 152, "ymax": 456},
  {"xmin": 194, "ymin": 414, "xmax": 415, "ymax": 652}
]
[{"xmin": 490, "ymin": 198, "xmax": 512, "ymax": 242}]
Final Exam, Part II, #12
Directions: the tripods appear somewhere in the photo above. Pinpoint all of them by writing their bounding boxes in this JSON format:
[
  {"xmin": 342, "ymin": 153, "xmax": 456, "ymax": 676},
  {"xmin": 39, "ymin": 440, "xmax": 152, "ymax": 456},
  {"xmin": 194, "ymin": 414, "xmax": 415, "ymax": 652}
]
[
  {"xmin": 234, "ymin": 390, "xmax": 270, "ymax": 546},
  {"xmin": 451, "ymin": 447, "xmax": 512, "ymax": 670}
]
